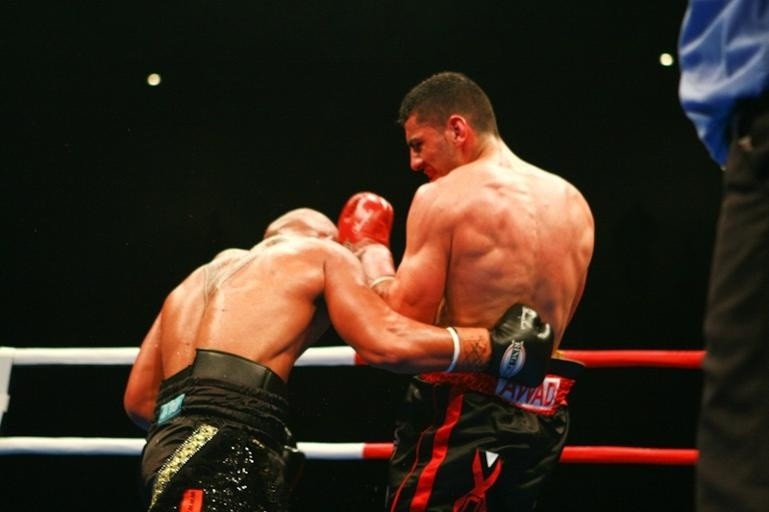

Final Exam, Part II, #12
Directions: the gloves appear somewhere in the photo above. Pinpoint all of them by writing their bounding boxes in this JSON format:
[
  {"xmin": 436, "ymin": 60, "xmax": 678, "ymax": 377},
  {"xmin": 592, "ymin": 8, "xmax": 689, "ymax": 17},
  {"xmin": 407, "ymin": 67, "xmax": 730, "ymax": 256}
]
[
  {"xmin": 447, "ymin": 303, "xmax": 554, "ymax": 389},
  {"xmin": 336, "ymin": 192, "xmax": 396, "ymax": 288}
]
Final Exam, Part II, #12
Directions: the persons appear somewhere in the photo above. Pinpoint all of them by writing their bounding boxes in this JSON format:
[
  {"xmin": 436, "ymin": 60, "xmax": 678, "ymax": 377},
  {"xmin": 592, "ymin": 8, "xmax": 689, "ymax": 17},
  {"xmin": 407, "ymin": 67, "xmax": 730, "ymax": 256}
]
[
  {"xmin": 124, "ymin": 207, "xmax": 554, "ymax": 510},
  {"xmin": 337, "ymin": 70, "xmax": 595, "ymax": 512},
  {"xmin": 679, "ymin": 2, "xmax": 769, "ymax": 511}
]
